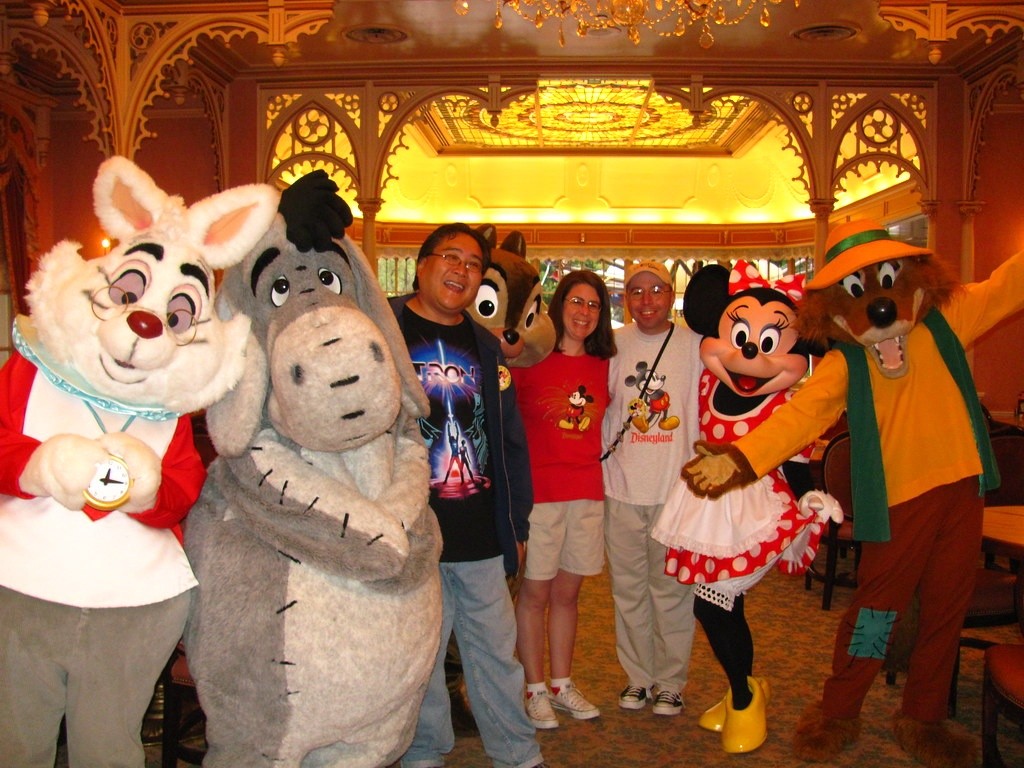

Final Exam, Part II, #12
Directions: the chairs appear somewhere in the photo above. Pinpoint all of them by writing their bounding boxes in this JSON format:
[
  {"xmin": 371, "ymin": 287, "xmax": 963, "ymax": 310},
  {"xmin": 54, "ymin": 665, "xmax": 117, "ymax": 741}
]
[{"xmin": 786, "ymin": 400, "xmax": 1024, "ymax": 768}]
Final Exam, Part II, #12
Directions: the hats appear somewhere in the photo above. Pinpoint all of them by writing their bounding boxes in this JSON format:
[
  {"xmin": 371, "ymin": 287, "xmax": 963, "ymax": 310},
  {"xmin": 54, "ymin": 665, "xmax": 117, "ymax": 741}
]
[{"xmin": 624, "ymin": 260, "xmax": 676, "ymax": 292}]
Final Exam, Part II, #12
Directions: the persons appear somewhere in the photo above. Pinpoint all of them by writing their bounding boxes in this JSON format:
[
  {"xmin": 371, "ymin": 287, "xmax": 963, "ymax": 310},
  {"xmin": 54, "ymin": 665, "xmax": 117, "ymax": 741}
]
[
  {"xmin": 509, "ymin": 270, "xmax": 619, "ymax": 728},
  {"xmin": 384, "ymin": 223, "xmax": 550, "ymax": 768},
  {"xmin": 601, "ymin": 262, "xmax": 707, "ymax": 715}
]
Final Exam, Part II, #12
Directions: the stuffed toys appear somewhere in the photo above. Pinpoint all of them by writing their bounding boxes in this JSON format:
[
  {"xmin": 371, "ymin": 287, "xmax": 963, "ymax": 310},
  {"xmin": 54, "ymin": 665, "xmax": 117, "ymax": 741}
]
[
  {"xmin": 440, "ymin": 224, "xmax": 555, "ymax": 736},
  {"xmin": 685, "ymin": 214, "xmax": 1024, "ymax": 768},
  {"xmin": 184, "ymin": 168, "xmax": 442, "ymax": 768},
  {"xmin": 0, "ymin": 154, "xmax": 282, "ymax": 768},
  {"xmin": 649, "ymin": 258, "xmax": 844, "ymax": 753}
]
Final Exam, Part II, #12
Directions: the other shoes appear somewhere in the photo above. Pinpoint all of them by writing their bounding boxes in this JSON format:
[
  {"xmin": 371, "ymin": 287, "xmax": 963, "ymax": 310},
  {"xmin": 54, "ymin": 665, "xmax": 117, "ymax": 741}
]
[{"xmin": 138, "ymin": 682, "xmax": 164, "ymax": 747}]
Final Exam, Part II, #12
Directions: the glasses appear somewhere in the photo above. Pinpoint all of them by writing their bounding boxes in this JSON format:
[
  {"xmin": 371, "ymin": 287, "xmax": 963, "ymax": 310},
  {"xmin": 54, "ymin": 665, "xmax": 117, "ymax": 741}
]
[
  {"xmin": 565, "ymin": 295, "xmax": 602, "ymax": 312},
  {"xmin": 625, "ymin": 285, "xmax": 673, "ymax": 299},
  {"xmin": 428, "ymin": 253, "xmax": 485, "ymax": 274}
]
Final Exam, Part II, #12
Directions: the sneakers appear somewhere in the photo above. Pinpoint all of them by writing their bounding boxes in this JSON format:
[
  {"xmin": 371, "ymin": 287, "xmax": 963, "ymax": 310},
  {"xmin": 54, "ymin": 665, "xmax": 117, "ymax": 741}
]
[
  {"xmin": 652, "ymin": 688, "xmax": 687, "ymax": 716},
  {"xmin": 618, "ymin": 682, "xmax": 654, "ymax": 710},
  {"xmin": 549, "ymin": 681, "xmax": 600, "ymax": 720},
  {"xmin": 523, "ymin": 689, "xmax": 559, "ymax": 729}
]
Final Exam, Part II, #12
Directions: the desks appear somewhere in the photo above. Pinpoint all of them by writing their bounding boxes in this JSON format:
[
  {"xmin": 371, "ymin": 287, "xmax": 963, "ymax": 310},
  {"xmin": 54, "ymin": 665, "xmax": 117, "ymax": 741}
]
[{"xmin": 977, "ymin": 505, "xmax": 1024, "ymax": 618}]
